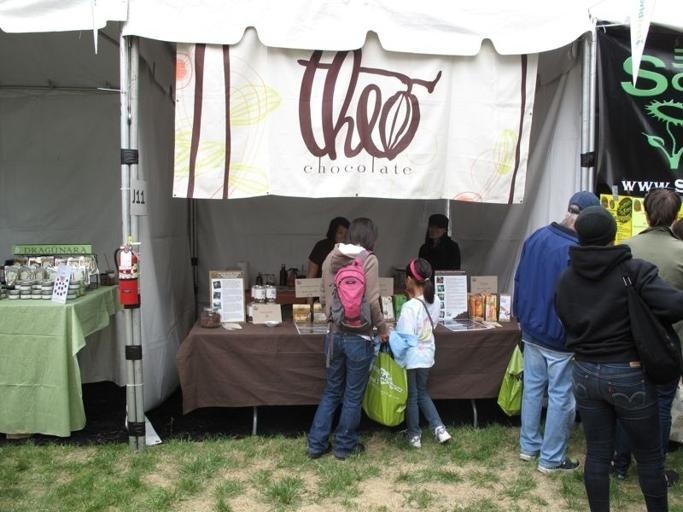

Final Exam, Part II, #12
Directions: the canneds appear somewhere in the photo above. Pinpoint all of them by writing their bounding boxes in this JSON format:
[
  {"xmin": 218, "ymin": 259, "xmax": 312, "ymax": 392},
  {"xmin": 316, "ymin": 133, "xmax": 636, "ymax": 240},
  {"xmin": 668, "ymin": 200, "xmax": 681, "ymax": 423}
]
[
  {"xmin": 201, "ymin": 308, "xmax": 221, "ymax": 327},
  {"xmin": 251, "ymin": 285, "xmax": 277, "ymax": 302}
]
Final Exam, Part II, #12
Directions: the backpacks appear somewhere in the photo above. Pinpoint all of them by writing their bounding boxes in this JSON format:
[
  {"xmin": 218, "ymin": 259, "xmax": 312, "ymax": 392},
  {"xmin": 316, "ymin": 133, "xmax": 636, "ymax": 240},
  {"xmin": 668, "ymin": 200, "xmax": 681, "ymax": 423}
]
[{"xmin": 331, "ymin": 251, "xmax": 374, "ymax": 331}]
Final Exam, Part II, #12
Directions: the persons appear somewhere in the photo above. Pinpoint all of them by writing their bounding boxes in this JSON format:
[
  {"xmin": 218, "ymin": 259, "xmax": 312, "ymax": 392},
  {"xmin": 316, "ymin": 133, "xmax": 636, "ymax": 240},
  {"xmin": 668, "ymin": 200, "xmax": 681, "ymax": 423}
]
[
  {"xmin": 418, "ymin": 214, "xmax": 461, "ymax": 287},
  {"xmin": 386, "ymin": 259, "xmax": 451, "ymax": 448},
  {"xmin": 611, "ymin": 188, "xmax": 683, "ymax": 487},
  {"xmin": 307, "ymin": 217, "xmax": 396, "ymax": 460},
  {"xmin": 552, "ymin": 205, "xmax": 683, "ymax": 512},
  {"xmin": 513, "ymin": 191, "xmax": 601, "ymax": 474},
  {"xmin": 307, "ymin": 216, "xmax": 350, "ymax": 305}
]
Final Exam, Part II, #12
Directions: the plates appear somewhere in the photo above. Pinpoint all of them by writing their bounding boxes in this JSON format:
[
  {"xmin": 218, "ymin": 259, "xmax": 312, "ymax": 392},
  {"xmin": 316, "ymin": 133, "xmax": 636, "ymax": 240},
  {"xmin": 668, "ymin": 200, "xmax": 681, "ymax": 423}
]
[{"xmin": 264, "ymin": 321, "xmax": 280, "ymax": 327}]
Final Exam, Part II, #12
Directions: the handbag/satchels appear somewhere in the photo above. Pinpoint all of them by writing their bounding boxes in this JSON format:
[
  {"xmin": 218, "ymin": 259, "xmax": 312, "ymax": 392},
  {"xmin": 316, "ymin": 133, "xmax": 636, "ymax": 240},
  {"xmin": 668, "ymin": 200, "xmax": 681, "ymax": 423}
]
[
  {"xmin": 627, "ymin": 286, "xmax": 681, "ymax": 382},
  {"xmin": 497, "ymin": 343, "xmax": 523, "ymax": 416},
  {"xmin": 363, "ymin": 340, "xmax": 408, "ymax": 427}
]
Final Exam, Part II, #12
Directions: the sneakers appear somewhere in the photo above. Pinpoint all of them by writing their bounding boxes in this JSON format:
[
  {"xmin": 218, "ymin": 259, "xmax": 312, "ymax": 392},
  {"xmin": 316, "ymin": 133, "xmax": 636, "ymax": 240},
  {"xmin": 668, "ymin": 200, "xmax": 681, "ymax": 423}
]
[
  {"xmin": 410, "ymin": 436, "xmax": 421, "ymax": 448},
  {"xmin": 432, "ymin": 425, "xmax": 451, "ymax": 443},
  {"xmin": 538, "ymin": 457, "xmax": 579, "ymax": 473}
]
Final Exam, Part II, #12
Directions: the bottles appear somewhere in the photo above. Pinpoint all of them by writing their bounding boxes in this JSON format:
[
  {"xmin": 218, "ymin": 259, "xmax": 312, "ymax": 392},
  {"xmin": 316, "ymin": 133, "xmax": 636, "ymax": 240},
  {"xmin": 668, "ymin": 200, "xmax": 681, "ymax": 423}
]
[
  {"xmin": 254, "ymin": 272, "xmax": 262, "ymax": 286},
  {"xmin": 279, "ymin": 263, "xmax": 297, "ymax": 288},
  {"xmin": 249, "ymin": 284, "xmax": 277, "ymax": 304},
  {"xmin": 99, "ymin": 269, "xmax": 115, "ymax": 287},
  {"xmin": 0, "ymin": 278, "xmax": 80, "ymax": 301},
  {"xmin": 199, "ymin": 306, "xmax": 220, "ymax": 329}
]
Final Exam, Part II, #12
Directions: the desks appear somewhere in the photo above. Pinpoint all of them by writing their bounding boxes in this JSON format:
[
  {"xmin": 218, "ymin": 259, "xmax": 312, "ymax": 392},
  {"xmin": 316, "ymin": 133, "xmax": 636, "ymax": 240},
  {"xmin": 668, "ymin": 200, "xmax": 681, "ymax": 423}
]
[
  {"xmin": 245, "ymin": 290, "xmax": 308, "ymax": 312},
  {"xmin": 176, "ymin": 309, "xmax": 523, "ymax": 436},
  {"xmin": 0, "ymin": 284, "xmax": 127, "ymax": 440}
]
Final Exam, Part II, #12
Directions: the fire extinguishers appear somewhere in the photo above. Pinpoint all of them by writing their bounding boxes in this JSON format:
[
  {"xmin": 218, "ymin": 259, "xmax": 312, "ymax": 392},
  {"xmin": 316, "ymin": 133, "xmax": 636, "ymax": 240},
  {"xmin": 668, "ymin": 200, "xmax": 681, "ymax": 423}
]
[{"xmin": 114, "ymin": 236, "xmax": 141, "ymax": 305}]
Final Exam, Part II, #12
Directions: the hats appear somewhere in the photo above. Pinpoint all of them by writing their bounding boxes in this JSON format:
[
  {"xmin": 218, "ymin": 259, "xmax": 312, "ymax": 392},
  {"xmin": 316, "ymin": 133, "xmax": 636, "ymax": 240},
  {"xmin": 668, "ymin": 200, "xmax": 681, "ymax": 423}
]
[
  {"xmin": 574, "ymin": 206, "xmax": 616, "ymax": 246},
  {"xmin": 568, "ymin": 191, "xmax": 600, "ymax": 213}
]
